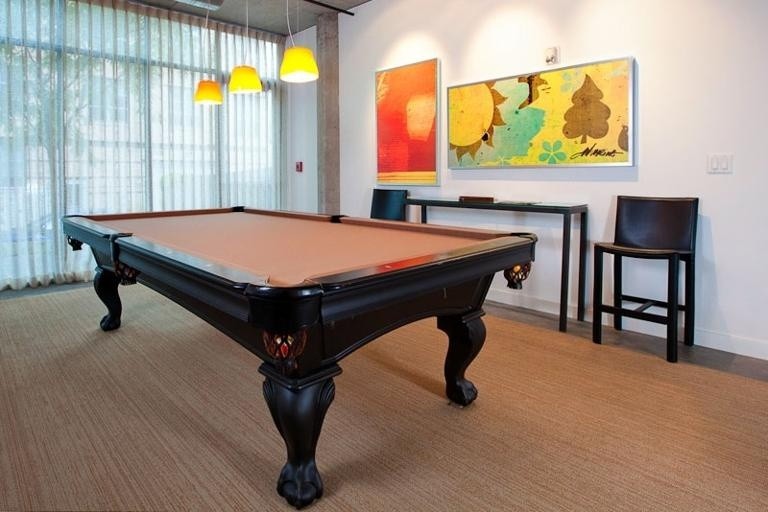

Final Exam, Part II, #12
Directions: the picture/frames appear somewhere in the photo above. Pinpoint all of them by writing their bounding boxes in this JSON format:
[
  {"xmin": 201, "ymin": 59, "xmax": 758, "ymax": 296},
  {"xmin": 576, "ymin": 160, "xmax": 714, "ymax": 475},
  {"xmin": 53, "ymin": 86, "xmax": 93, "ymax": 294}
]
[
  {"xmin": 373, "ymin": 55, "xmax": 436, "ymax": 189},
  {"xmin": 448, "ymin": 56, "xmax": 636, "ymax": 171}
]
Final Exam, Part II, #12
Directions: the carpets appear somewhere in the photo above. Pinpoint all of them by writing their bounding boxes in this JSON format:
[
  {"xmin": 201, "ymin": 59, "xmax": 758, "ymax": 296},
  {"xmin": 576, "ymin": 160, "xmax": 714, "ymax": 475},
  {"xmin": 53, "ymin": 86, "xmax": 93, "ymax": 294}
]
[{"xmin": 0, "ymin": 288, "xmax": 768, "ymax": 512}]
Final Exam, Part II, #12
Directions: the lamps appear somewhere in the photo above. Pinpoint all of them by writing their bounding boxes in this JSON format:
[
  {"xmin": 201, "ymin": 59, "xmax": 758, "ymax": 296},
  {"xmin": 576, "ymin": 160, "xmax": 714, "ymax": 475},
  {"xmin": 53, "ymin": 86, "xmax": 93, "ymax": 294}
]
[
  {"xmin": 227, "ymin": 0, "xmax": 263, "ymax": 94},
  {"xmin": 193, "ymin": 0, "xmax": 225, "ymax": 107},
  {"xmin": 279, "ymin": 1, "xmax": 319, "ymax": 84}
]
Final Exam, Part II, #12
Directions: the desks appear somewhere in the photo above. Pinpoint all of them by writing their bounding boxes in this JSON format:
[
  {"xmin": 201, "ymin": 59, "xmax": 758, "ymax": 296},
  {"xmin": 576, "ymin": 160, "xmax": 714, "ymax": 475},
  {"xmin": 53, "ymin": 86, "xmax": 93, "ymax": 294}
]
[
  {"xmin": 401, "ymin": 196, "xmax": 588, "ymax": 332},
  {"xmin": 62, "ymin": 205, "xmax": 536, "ymax": 510}
]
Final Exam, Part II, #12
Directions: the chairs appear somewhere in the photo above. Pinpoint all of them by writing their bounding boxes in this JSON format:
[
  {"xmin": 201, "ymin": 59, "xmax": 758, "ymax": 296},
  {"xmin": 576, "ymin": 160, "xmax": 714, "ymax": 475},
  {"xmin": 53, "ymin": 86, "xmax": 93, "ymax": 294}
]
[
  {"xmin": 592, "ymin": 195, "xmax": 698, "ymax": 363},
  {"xmin": 369, "ymin": 189, "xmax": 409, "ymax": 222}
]
